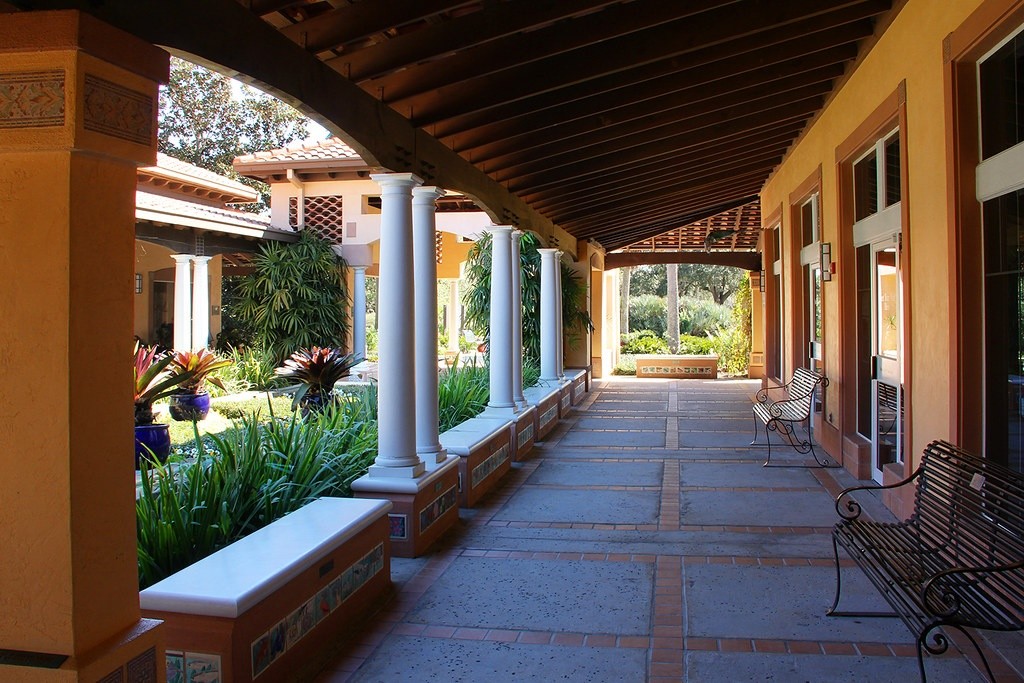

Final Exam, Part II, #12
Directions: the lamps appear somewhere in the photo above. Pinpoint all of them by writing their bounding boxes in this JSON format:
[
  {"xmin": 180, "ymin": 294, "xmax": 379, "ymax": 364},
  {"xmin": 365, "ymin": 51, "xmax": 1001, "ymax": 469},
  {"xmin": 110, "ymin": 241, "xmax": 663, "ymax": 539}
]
[
  {"xmin": 819, "ymin": 242, "xmax": 831, "ymax": 282},
  {"xmin": 135, "ymin": 272, "xmax": 142, "ymax": 294},
  {"xmin": 759, "ymin": 270, "xmax": 765, "ymax": 292}
]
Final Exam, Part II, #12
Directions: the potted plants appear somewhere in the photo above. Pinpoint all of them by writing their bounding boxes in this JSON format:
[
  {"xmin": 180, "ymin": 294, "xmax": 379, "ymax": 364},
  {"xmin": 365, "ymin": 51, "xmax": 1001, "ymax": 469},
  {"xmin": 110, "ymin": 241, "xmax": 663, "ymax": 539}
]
[
  {"xmin": 274, "ymin": 342, "xmax": 366, "ymax": 428},
  {"xmin": 133, "ymin": 340, "xmax": 198, "ymax": 472},
  {"xmin": 154, "ymin": 346, "xmax": 234, "ymax": 420}
]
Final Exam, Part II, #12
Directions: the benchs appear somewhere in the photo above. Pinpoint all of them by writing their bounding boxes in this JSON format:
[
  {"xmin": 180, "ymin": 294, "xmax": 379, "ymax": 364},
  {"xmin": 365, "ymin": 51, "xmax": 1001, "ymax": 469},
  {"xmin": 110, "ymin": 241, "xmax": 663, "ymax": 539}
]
[
  {"xmin": 523, "ymin": 387, "xmax": 560, "ymax": 441},
  {"xmin": 137, "ymin": 493, "xmax": 396, "ymax": 683},
  {"xmin": 878, "ymin": 380, "xmax": 903, "ymax": 436},
  {"xmin": 562, "ymin": 370, "xmax": 587, "ymax": 405},
  {"xmin": 435, "ymin": 416, "xmax": 515, "ymax": 508},
  {"xmin": 748, "ymin": 365, "xmax": 830, "ymax": 469},
  {"xmin": 825, "ymin": 437, "xmax": 1024, "ymax": 683}
]
